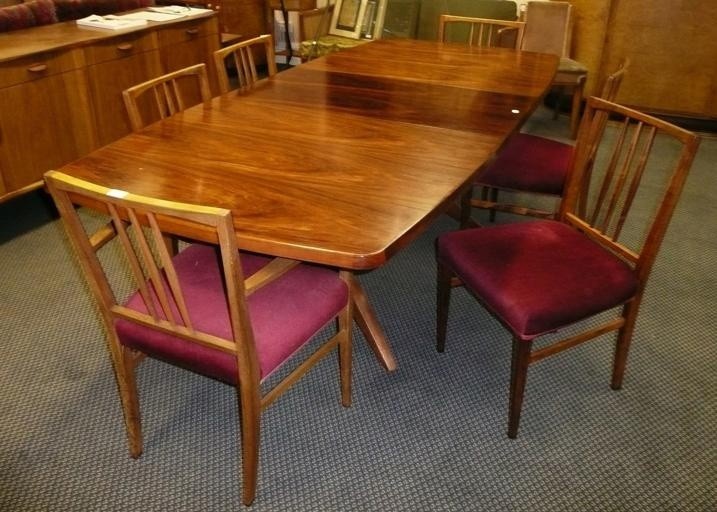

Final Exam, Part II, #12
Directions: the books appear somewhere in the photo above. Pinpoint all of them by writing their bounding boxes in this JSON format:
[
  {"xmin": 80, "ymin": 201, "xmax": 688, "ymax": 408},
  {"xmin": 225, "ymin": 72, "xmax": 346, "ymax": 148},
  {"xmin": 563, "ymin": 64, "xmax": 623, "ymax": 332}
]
[{"xmin": 76, "ymin": 4, "xmax": 215, "ymax": 31}]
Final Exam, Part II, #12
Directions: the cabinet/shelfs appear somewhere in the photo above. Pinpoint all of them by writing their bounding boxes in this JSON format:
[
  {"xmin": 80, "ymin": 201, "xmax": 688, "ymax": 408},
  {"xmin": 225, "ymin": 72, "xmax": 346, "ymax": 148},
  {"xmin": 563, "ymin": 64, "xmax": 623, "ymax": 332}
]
[{"xmin": 0, "ymin": 5, "xmax": 223, "ymax": 205}]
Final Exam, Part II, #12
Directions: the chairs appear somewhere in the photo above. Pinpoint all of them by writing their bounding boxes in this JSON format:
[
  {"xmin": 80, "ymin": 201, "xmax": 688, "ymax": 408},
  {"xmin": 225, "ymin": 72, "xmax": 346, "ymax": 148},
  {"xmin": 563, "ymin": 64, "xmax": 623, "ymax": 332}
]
[
  {"xmin": 518, "ymin": 1, "xmax": 589, "ymax": 139},
  {"xmin": 213, "ymin": 33, "xmax": 281, "ymax": 94},
  {"xmin": 437, "ymin": 13, "xmax": 529, "ymax": 51},
  {"xmin": 120, "ymin": 62, "xmax": 215, "ymax": 130},
  {"xmin": 433, "ymin": 93, "xmax": 703, "ymax": 443},
  {"xmin": 457, "ymin": 52, "xmax": 634, "ymax": 230},
  {"xmin": 41, "ymin": 168, "xmax": 356, "ymax": 506}
]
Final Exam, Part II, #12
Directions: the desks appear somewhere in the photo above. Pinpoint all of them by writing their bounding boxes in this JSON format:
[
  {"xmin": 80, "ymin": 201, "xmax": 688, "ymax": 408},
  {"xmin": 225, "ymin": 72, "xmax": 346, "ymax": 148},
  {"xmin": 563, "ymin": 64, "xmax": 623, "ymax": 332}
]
[
  {"xmin": 47, "ymin": 35, "xmax": 574, "ymax": 372},
  {"xmin": 297, "ymin": 34, "xmax": 371, "ymax": 64},
  {"xmin": 221, "ymin": 31, "xmax": 242, "ymax": 46}
]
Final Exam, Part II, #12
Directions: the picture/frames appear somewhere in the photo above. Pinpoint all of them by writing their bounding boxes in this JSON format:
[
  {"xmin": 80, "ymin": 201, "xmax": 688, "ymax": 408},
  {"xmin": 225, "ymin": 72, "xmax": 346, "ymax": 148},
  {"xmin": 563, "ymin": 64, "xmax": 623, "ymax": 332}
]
[
  {"xmin": 359, "ymin": 1, "xmax": 388, "ymax": 39},
  {"xmin": 326, "ymin": 0, "xmax": 367, "ymax": 38}
]
